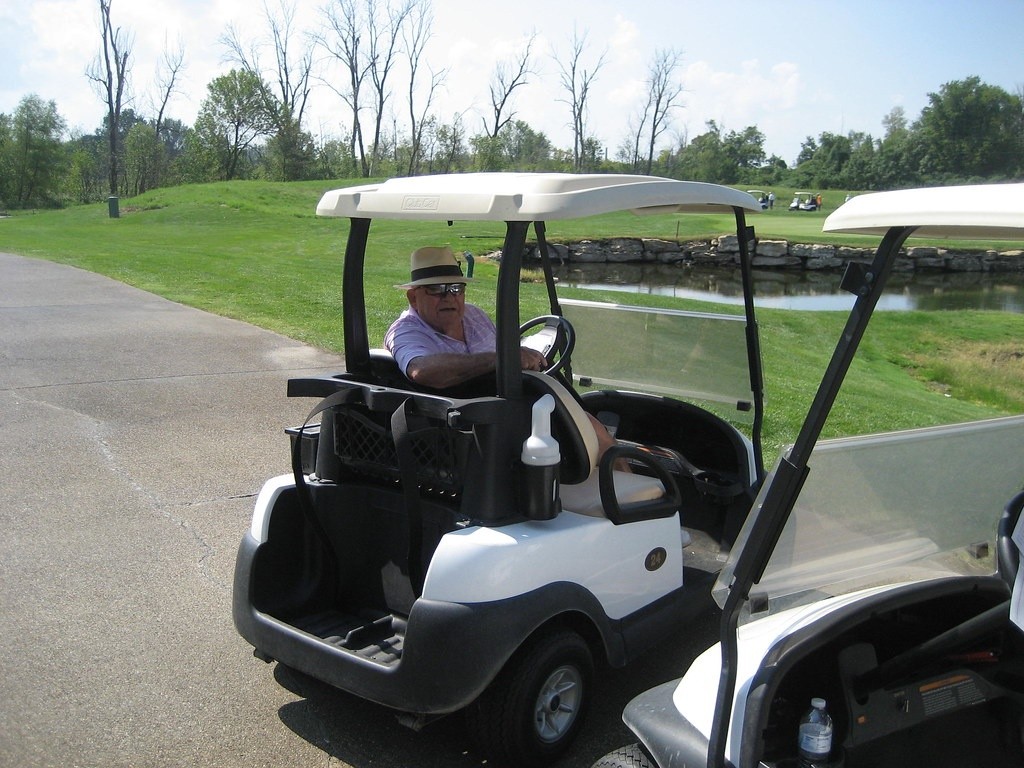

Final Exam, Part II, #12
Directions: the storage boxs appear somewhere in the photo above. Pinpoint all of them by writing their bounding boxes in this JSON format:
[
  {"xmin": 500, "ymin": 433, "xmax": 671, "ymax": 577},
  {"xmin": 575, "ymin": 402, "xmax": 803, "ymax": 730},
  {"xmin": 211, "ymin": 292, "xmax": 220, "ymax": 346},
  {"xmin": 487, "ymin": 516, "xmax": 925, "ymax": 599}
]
[{"xmin": 283, "ymin": 424, "xmax": 321, "ymax": 474}]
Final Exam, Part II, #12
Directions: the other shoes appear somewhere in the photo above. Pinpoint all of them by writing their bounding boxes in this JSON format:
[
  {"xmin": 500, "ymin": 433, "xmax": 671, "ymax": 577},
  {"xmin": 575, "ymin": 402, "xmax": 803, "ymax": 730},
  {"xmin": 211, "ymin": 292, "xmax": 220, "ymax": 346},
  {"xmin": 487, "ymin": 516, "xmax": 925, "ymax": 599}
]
[{"xmin": 681, "ymin": 529, "xmax": 690, "ymax": 548}]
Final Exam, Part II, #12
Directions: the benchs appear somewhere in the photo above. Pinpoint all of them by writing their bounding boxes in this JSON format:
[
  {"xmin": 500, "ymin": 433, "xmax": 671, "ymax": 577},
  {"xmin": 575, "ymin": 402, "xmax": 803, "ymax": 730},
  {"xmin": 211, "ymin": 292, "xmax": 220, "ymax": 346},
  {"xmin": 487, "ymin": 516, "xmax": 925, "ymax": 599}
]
[{"xmin": 369, "ymin": 349, "xmax": 682, "ymax": 526}]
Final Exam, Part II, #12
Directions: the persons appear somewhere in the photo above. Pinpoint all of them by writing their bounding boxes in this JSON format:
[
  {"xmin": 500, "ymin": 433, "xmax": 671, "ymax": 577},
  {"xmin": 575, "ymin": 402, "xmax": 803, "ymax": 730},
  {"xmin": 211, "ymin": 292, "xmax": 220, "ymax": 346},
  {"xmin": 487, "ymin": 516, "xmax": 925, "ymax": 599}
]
[
  {"xmin": 384, "ymin": 248, "xmax": 691, "ymax": 549},
  {"xmin": 767, "ymin": 191, "xmax": 776, "ymax": 210},
  {"xmin": 817, "ymin": 193, "xmax": 822, "ymax": 210},
  {"xmin": 844, "ymin": 195, "xmax": 852, "ymax": 203}
]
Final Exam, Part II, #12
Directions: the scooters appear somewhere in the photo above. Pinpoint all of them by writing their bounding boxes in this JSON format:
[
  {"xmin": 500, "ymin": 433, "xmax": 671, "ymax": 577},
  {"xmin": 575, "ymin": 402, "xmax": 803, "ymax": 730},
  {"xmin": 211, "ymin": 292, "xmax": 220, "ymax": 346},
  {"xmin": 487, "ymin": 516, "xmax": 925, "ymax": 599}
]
[
  {"xmin": 789, "ymin": 192, "xmax": 817, "ymax": 212},
  {"xmin": 589, "ymin": 183, "xmax": 1024, "ymax": 768},
  {"xmin": 747, "ymin": 190, "xmax": 769, "ymax": 210},
  {"xmin": 231, "ymin": 172, "xmax": 768, "ymax": 768}
]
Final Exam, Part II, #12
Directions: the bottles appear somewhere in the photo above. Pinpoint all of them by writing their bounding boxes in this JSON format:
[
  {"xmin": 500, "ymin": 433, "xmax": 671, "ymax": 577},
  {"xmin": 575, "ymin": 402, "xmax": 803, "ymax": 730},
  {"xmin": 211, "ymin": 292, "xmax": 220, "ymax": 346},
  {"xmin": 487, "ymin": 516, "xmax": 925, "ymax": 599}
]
[{"xmin": 798, "ymin": 698, "xmax": 833, "ymax": 768}]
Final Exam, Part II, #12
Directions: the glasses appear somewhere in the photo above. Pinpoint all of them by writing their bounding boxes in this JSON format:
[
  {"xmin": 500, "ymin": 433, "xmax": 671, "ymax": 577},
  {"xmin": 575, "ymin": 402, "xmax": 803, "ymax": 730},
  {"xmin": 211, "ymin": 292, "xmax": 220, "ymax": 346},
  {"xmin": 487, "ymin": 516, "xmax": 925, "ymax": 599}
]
[{"xmin": 414, "ymin": 283, "xmax": 465, "ymax": 296}]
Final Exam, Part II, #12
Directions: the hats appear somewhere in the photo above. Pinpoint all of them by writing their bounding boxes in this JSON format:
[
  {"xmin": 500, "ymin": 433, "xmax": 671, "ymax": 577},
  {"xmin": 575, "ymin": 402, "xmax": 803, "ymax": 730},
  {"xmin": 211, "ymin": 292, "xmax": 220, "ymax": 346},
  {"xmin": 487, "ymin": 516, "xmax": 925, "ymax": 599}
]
[{"xmin": 392, "ymin": 246, "xmax": 476, "ymax": 289}]
